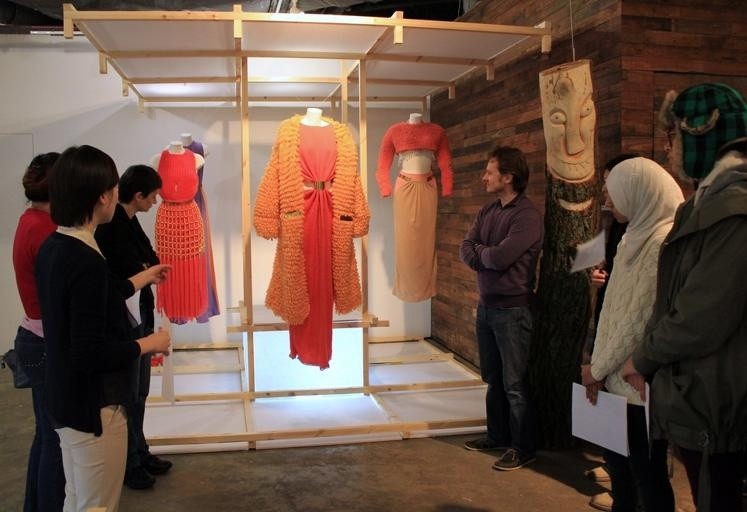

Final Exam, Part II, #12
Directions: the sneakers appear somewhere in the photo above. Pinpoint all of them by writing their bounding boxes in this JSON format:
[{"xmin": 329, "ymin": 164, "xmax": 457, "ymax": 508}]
[
  {"xmin": 589, "ymin": 491, "xmax": 613, "ymax": 511},
  {"xmin": 125, "ymin": 463, "xmax": 155, "ymax": 489},
  {"xmin": 464, "ymin": 439, "xmax": 509, "ymax": 450},
  {"xmin": 142, "ymin": 454, "xmax": 173, "ymax": 473},
  {"xmin": 492, "ymin": 447, "xmax": 535, "ymax": 471},
  {"xmin": 587, "ymin": 464, "xmax": 611, "ymax": 482}
]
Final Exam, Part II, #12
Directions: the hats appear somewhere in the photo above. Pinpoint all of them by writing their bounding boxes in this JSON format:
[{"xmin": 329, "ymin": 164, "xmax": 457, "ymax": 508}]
[{"xmin": 658, "ymin": 82, "xmax": 747, "ymax": 183}]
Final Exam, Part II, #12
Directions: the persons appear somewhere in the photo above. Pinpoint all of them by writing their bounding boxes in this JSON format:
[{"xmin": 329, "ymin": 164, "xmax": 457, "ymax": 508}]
[
  {"xmin": 580, "ymin": 155, "xmax": 677, "ymax": 511},
  {"xmin": 620, "ymin": 83, "xmax": 746, "ymax": 512},
  {"xmin": 460, "ymin": 145, "xmax": 545, "ymax": 472},
  {"xmin": 12, "ymin": 151, "xmax": 67, "ymax": 512},
  {"xmin": 35, "ymin": 144, "xmax": 172, "ymax": 512},
  {"xmin": 95, "ymin": 167, "xmax": 173, "ymax": 488}
]
